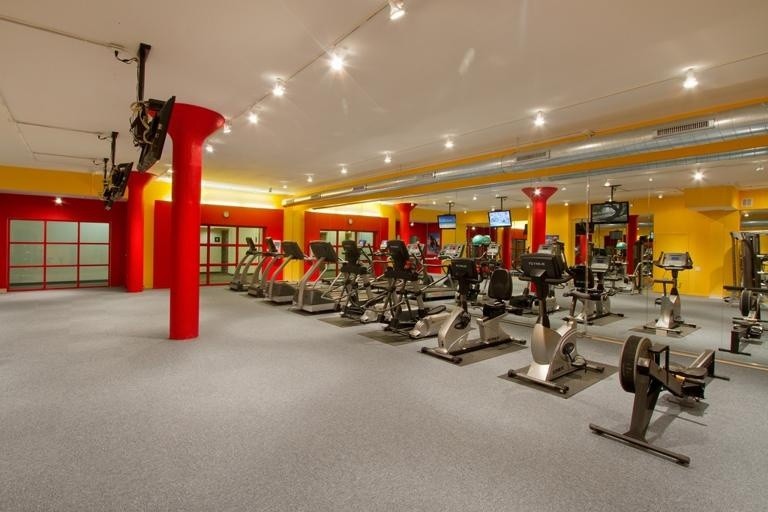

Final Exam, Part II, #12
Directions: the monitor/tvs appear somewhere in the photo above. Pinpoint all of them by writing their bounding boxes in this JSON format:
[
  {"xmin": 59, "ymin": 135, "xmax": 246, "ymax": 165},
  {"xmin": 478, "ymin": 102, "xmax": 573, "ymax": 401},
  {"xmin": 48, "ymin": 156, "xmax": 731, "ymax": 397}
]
[
  {"xmin": 575, "ymin": 201, "xmax": 693, "ymax": 273},
  {"xmin": 103, "ymin": 187, "xmax": 119, "ymax": 212},
  {"xmin": 281, "ymin": 241, "xmax": 304, "ymax": 259},
  {"xmin": 488, "ymin": 210, "xmax": 512, "ymax": 227},
  {"xmin": 309, "ymin": 239, "xmax": 426, "ymax": 263},
  {"xmin": 265, "ymin": 237, "xmax": 278, "ymax": 253},
  {"xmin": 437, "ymin": 214, "xmax": 456, "ymax": 229},
  {"xmin": 245, "ymin": 237, "xmax": 257, "ymax": 251},
  {"xmin": 109, "ymin": 161, "xmax": 134, "ymax": 198},
  {"xmin": 136, "ymin": 95, "xmax": 176, "ymax": 174},
  {"xmin": 438, "ymin": 243, "xmax": 563, "ymax": 284}
]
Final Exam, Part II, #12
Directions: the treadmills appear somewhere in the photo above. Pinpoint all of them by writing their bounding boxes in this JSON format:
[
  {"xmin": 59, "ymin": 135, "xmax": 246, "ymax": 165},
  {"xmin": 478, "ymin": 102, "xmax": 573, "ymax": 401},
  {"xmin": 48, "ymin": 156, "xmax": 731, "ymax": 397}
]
[
  {"xmin": 322, "ymin": 240, "xmax": 468, "ymax": 299},
  {"xmin": 228, "ymin": 237, "xmax": 384, "ymax": 312}
]
